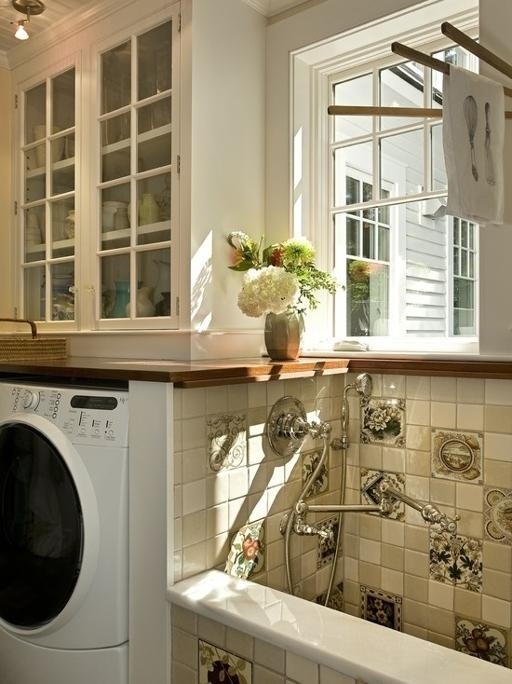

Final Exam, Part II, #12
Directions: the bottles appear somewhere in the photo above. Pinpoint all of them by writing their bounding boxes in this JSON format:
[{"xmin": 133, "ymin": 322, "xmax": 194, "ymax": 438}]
[{"xmin": 139, "ymin": 194, "xmax": 161, "ymax": 227}]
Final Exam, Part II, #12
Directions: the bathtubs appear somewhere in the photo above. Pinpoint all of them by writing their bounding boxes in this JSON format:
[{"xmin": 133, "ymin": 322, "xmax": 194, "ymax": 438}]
[{"xmin": 170, "ymin": 552, "xmax": 510, "ymax": 682}]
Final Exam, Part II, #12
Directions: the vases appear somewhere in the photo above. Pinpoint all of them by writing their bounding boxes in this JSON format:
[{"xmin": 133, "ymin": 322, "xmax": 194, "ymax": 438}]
[{"xmin": 263, "ymin": 309, "xmax": 301, "ymax": 363}]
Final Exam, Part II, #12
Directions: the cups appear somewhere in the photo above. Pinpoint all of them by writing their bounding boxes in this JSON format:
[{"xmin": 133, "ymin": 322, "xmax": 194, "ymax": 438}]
[
  {"xmin": 33, "ymin": 125, "xmax": 66, "ymax": 168},
  {"xmin": 102, "ymin": 201, "xmax": 130, "ymax": 234}
]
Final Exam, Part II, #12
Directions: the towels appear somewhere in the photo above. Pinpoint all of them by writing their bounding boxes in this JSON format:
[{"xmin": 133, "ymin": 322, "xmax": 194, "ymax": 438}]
[{"xmin": 422, "ymin": 62, "xmax": 504, "ymax": 229}]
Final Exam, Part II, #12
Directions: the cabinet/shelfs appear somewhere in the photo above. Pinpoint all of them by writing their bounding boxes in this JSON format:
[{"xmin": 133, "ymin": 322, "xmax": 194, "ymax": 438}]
[{"xmin": 10, "ymin": 0, "xmax": 267, "ymax": 357}]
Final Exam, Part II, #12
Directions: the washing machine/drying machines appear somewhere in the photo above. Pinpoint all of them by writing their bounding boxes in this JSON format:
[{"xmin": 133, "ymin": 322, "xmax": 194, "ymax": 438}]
[{"xmin": 0, "ymin": 376, "xmax": 185, "ymax": 683}]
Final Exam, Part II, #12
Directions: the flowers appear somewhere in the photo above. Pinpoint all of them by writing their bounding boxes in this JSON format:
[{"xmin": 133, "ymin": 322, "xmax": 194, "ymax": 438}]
[{"xmin": 227, "ymin": 227, "xmax": 343, "ymax": 319}]
